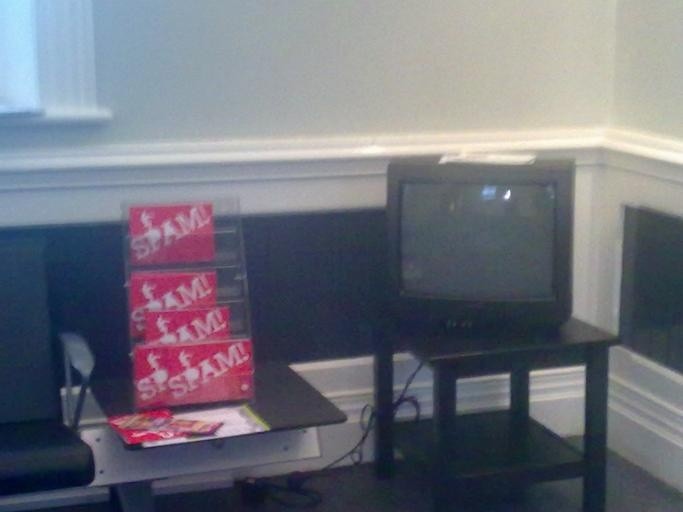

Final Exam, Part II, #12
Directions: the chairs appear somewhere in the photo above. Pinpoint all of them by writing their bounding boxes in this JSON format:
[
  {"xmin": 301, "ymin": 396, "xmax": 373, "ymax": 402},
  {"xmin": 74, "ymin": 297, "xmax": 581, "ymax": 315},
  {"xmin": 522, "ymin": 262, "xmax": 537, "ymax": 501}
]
[{"xmin": 1, "ymin": 234, "xmax": 96, "ymax": 500}]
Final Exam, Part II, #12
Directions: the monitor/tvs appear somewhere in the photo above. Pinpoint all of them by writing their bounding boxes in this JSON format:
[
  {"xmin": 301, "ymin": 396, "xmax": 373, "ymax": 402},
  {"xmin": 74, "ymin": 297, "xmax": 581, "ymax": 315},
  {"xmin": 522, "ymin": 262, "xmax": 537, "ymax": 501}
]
[{"xmin": 384, "ymin": 152, "xmax": 577, "ymax": 338}]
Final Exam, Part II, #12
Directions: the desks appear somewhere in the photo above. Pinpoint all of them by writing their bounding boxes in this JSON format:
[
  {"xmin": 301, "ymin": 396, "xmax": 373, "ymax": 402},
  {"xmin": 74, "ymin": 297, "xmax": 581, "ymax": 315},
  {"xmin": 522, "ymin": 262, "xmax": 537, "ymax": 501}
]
[
  {"xmin": 373, "ymin": 315, "xmax": 626, "ymax": 512},
  {"xmin": 79, "ymin": 356, "xmax": 348, "ymax": 512}
]
